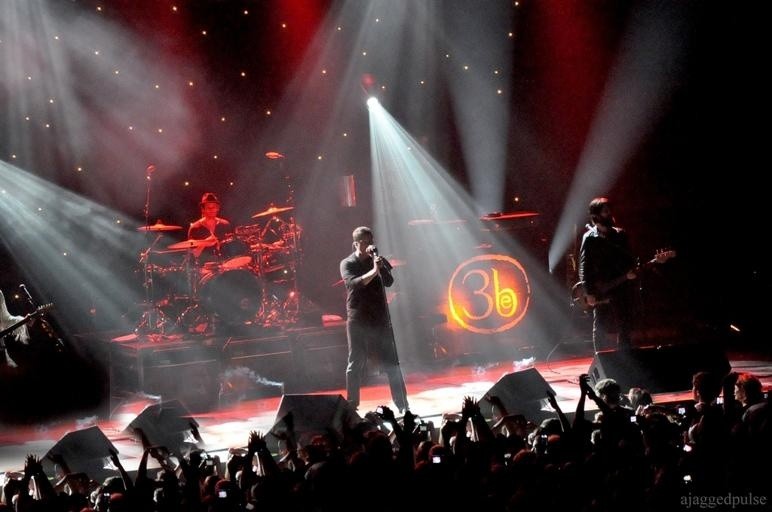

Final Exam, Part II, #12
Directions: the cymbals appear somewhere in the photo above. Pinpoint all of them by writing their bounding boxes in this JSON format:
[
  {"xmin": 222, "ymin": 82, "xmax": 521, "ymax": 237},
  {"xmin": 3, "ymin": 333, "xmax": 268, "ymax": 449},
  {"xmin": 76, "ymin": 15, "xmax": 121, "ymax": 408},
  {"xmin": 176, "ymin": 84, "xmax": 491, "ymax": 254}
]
[
  {"xmin": 168, "ymin": 240, "xmax": 218, "ymax": 249},
  {"xmin": 136, "ymin": 224, "xmax": 183, "ymax": 231},
  {"xmin": 251, "ymin": 207, "xmax": 294, "ymax": 219}
]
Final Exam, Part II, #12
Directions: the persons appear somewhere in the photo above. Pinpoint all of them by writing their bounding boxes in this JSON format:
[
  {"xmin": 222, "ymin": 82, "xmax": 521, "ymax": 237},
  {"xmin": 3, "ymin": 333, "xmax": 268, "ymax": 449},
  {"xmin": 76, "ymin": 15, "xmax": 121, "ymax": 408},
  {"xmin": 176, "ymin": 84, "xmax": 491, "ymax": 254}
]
[
  {"xmin": 187, "ymin": 190, "xmax": 237, "ymax": 266},
  {"xmin": 69, "ymin": 309, "xmax": 112, "ymax": 369},
  {"xmin": 338, "ymin": 226, "xmax": 410, "ymax": 422},
  {"xmin": 577, "ymin": 196, "xmax": 667, "ymax": 357},
  {"xmin": 2, "ymin": 370, "xmax": 769, "ymax": 511},
  {"xmin": 0, "ymin": 290, "xmax": 37, "ymax": 371}
]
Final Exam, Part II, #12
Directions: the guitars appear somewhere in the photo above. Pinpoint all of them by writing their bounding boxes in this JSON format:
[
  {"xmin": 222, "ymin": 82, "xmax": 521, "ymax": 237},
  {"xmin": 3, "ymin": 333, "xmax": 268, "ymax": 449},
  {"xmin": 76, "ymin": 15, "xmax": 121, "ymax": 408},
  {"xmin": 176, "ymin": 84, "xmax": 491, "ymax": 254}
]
[
  {"xmin": 1, "ymin": 303, "xmax": 55, "ymax": 339},
  {"xmin": 571, "ymin": 246, "xmax": 676, "ymax": 314}
]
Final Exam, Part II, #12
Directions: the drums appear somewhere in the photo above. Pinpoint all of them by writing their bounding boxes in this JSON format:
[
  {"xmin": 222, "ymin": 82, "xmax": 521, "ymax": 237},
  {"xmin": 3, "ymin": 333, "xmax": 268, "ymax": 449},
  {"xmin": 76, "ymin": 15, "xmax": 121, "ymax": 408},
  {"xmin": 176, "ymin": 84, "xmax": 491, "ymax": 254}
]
[
  {"xmin": 194, "ymin": 269, "xmax": 262, "ymax": 321},
  {"xmin": 218, "ymin": 238, "xmax": 253, "ymax": 268}
]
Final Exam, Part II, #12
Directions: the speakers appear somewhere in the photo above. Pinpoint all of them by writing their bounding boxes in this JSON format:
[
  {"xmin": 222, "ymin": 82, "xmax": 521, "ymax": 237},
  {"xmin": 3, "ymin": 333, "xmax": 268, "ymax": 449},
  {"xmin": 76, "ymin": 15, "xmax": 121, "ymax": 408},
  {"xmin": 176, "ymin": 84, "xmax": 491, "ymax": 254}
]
[
  {"xmin": 586, "ymin": 348, "xmax": 672, "ymax": 395},
  {"xmin": 123, "ymin": 400, "xmax": 200, "ymax": 452},
  {"xmin": 40, "ymin": 426, "xmax": 118, "ymax": 476},
  {"xmin": 274, "ymin": 395, "xmax": 362, "ymax": 436},
  {"xmin": 478, "ymin": 368, "xmax": 557, "ymax": 418},
  {"xmin": 260, "ymin": 405, "xmax": 342, "ymax": 455},
  {"xmin": 665, "ymin": 344, "xmax": 731, "ymax": 391}
]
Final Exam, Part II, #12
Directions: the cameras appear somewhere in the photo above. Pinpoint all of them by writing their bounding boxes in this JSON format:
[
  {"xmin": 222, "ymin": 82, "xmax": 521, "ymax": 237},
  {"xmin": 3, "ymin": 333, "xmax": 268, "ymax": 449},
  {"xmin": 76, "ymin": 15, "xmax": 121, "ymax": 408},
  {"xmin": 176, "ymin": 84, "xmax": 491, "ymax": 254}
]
[
  {"xmin": 716, "ymin": 396, "xmax": 725, "ymax": 404},
  {"xmin": 431, "ymin": 455, "xmax": 443, "ymax": 465},
  {"xmin": 376, "ymin": 406, "xmax": 383, "ymax": 415},
  {"xmin": 418, "ymin": 424, "xmax": 428, "ymax": 431},
  {"xmin": 204, "ymin": 459, "xmax": 216, "ymax": 466},
  {"xmin": 630, "ymin": 416, "xmax": 637, "ymax": 422},
  {"xmin": 677, "ymin": 407, "xmax": 686, "ymax": 415}
]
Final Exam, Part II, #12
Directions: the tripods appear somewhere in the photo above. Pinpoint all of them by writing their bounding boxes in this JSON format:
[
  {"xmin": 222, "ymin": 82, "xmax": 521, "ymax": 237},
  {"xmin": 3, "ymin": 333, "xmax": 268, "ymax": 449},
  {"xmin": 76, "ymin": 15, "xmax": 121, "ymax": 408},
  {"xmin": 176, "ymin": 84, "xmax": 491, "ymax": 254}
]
[{"xmin": 131, "ymin": 174, "xmax": 325, "ymax": 343}]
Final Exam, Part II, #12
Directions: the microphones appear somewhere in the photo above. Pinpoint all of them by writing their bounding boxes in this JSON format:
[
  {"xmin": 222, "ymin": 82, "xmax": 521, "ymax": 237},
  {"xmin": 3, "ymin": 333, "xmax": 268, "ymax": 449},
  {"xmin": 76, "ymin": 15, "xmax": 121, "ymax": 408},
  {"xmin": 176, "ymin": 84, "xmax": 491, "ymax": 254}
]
[
  {"xmin": 146, "ymin": 165, "xmax": 156, "ymax": 173},
  {"xmin": 369, "ymin": 248, "xmax": 381, "ymax": 265},
  {"xmin": 20, "ymin": 284, "xmax": 33, "ymax": 299},
  {"xmin": 264, "ymin": 152, "xmax": 285, "ymax": 159}
]
